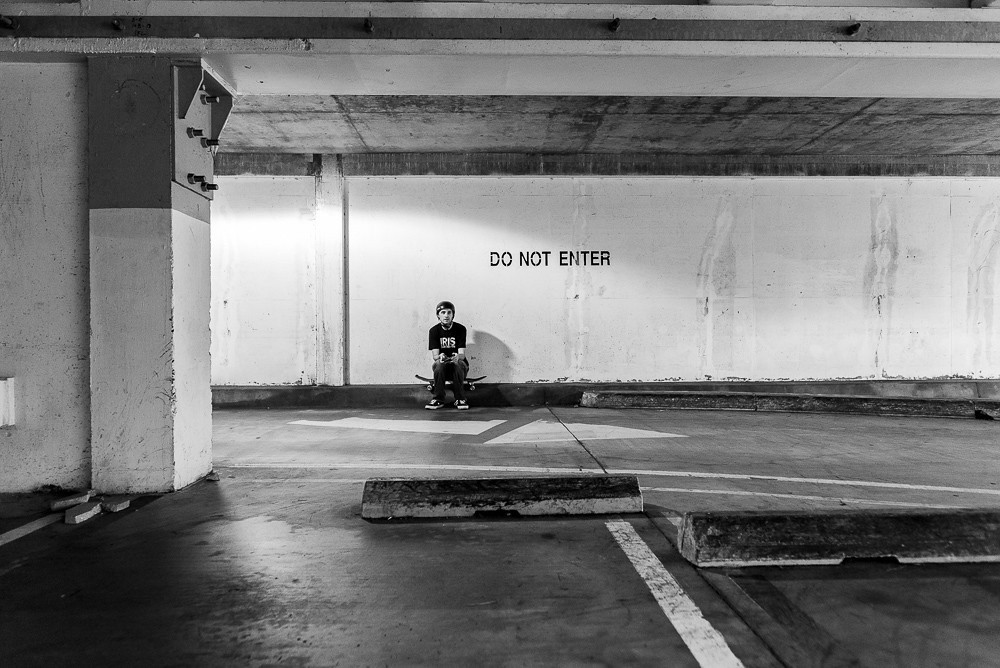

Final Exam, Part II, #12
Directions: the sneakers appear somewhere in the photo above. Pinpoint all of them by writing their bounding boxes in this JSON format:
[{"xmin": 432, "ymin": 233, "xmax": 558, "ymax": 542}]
[
  {"xmin": 453, "ymin": 399, "xmax": 470, "ymax": 409},
  {"xmin": 425, "ymin": 398, "xmax": 445, "ymax": 410}
]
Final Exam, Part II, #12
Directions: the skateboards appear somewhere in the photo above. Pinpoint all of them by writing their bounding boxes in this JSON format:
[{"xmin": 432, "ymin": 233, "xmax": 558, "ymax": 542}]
[{"xmin": 415, "ymin": 375, "xmax": 487, "ymax": 391}]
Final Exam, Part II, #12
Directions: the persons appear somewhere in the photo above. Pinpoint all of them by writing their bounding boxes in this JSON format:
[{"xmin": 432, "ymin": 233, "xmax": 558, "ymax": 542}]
[{"xmin": 423, "ymin": 300, "xmax": 470, "ymax": 411}]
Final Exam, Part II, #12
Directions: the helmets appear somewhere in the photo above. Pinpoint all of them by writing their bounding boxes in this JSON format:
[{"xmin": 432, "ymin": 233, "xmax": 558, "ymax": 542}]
[{"xmin": 436, "ymin": 301, "xmax": 455, "ymax": 320}]
